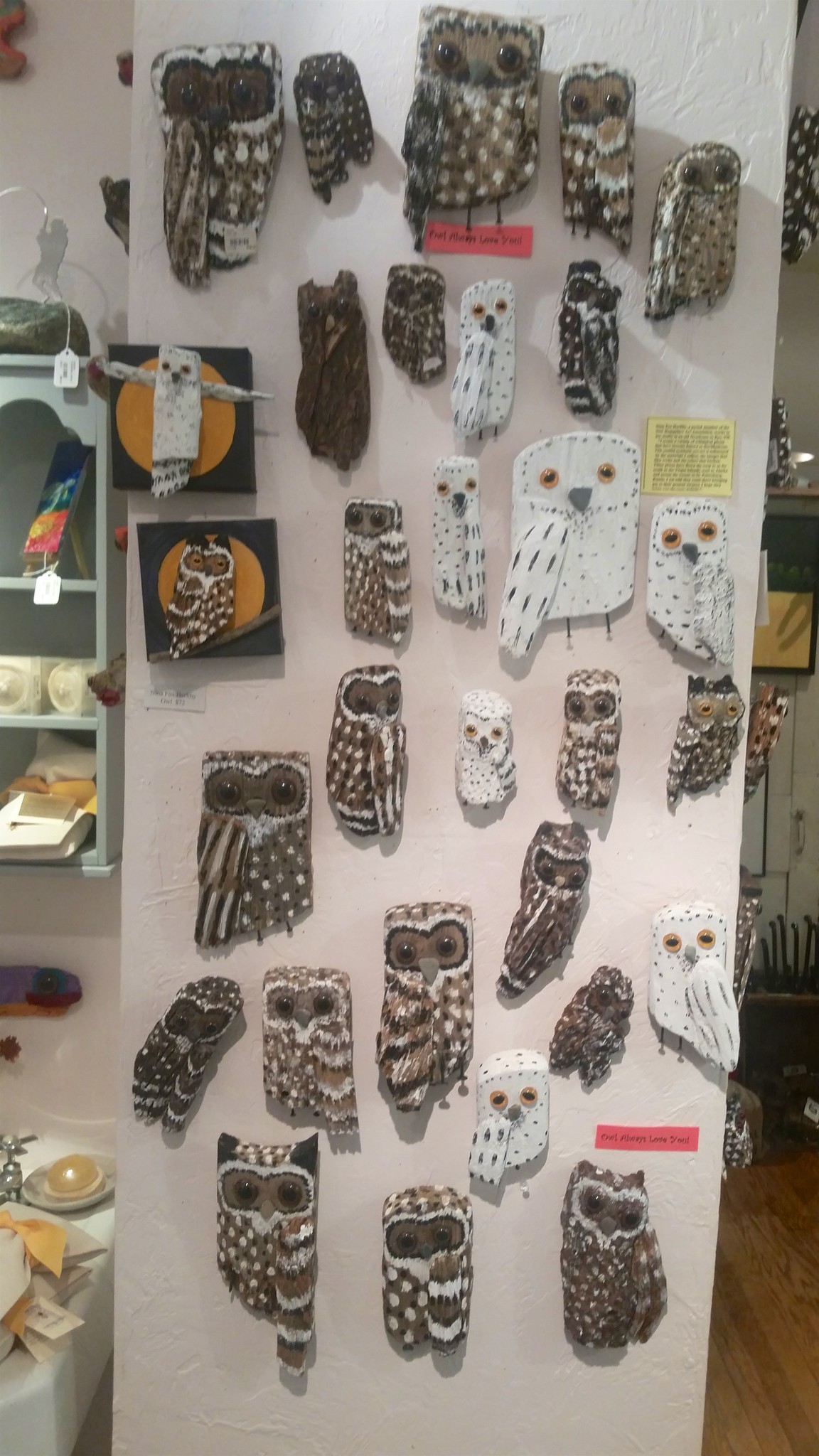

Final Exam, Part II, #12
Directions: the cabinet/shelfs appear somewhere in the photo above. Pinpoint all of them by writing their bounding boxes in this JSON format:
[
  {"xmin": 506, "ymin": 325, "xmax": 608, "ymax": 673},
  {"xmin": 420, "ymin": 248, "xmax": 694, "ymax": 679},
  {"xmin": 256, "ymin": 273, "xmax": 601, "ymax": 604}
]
[{"xmin": 0, "ymin": 351, "xmax": 132, "ymax": 883}]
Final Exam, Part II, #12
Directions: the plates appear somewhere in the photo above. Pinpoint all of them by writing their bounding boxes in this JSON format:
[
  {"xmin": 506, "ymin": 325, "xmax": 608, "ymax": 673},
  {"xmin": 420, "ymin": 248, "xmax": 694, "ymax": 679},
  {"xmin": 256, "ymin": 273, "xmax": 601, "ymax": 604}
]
[{"xmin": 22, "ymin": 1154, "xmax": 117, "ymax": 1213}]
